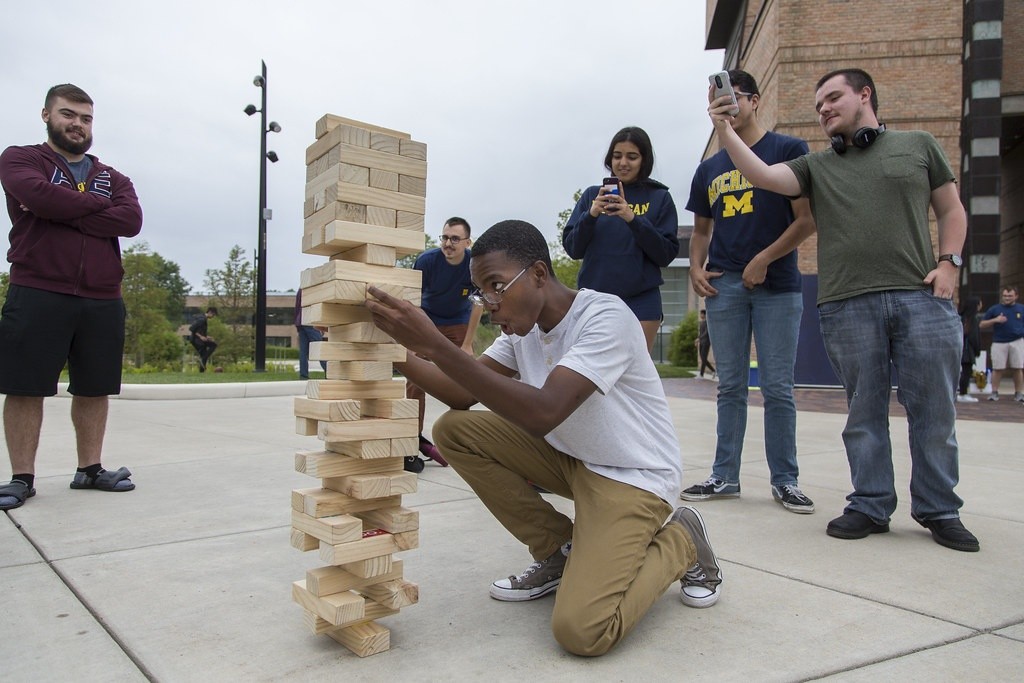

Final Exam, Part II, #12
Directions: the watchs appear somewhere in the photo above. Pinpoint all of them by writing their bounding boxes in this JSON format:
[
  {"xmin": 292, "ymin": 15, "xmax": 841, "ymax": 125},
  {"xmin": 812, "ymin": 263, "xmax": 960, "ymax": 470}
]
[{"xmin": 938, "ymin": 254, "xmax": 964, "ymax": 269}]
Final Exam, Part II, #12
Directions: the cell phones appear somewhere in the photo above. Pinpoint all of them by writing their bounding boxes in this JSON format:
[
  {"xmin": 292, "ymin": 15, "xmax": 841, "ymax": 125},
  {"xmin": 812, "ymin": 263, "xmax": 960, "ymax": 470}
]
[
  {"xmin": 709, "ymin": 71, "xmax": 739, "ymax": 115},
  {"xmin": 603, "ymin": 177, "xmax": 620, "ymax": 212}
]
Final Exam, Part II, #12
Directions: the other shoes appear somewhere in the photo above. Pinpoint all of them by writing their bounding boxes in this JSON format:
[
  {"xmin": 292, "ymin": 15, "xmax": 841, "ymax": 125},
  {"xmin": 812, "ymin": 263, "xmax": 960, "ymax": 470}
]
[
  {"xmin": 826, "ymin": 511, "xmax": 890, "ymax": 539},
  {"xmin": 1013, "ymin": 392, "xmax": 1024, "ymax": 402},
  {"xmin": 986, "ymin": 393, "xmax": 1000, "ymax": 401},
  {"xmin": 957, "ymin": 394, "xmax": 979, "ymax": 403},
  {"xmin": 911, "ymin": 512, "xmax": 981, "ymax": 551}
]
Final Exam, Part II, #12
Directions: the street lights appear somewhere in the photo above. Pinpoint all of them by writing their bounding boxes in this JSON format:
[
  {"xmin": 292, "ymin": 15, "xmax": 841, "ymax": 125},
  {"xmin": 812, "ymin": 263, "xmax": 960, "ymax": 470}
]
[{"xmin": 242, "ymin": 59, "xmax": 281, "ymax": 370}]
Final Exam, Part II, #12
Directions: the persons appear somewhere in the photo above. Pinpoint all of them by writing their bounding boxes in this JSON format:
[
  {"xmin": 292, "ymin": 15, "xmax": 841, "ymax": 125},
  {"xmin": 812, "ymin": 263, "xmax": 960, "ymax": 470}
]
[
  {"xmin": 362, "ymin": 220, "xmax": 723, "ymax": 658},
  {"xmin": 403, "ymin": 216, "xmax": 483, "ymax": 438},
  {"xmin": 188, "ymin": 306, "xmax": 218, "ymax": 374},
  {"xmin": 686, "ymin": 70, "xmax": 816, "ymax": 517},
  {"xmin": 294, "ymin": 285, "xmax": 327, "ymax": 380},
  {"xmin": 704, "ymin": 68, "xmax": 980, "ymax": 553},
  {"xmin": 957, "ymin": 296, "xmax": 979, "ymax": 404},
  {"xmin": 561, "ymin": 126, "xmax": 680, "ymax": 354},
  {"xmin": 1, "ymin": 83, "xmax": 143, "ymax": 510},
  {"xmin": 979, "ymin": 285, "xmax": 1024, "ymax": 402}
]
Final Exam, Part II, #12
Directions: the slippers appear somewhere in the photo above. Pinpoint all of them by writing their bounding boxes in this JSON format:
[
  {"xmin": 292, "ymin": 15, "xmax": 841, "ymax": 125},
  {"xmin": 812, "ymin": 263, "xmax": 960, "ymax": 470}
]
[
  {"xmin": 69, "ymin": 467, "xmax": 136, "ymax": 492},
  {"xmin": 0, "ymin": 483, "xmax": 37, "ymax": 509}
]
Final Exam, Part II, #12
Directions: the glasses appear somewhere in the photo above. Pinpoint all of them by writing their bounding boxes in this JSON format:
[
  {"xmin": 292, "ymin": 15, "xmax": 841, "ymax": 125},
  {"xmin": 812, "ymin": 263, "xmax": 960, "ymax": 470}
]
[
  {"xmin": 734, "ymin": 90, "xmax": 753, "ymax": 100},
  {"xmin": 438, "ymin": 234, "xmax": 467, "ymax": 244},
  {"xmin": 466, "ymin": 265, "xmax": 531, "ymax": 306}
]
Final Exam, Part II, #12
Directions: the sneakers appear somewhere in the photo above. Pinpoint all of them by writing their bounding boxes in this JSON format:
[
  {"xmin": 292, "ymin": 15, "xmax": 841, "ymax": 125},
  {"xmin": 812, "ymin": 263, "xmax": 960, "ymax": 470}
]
[
  {"xmin": 679, "ymin": 477, "xmax": 741, "ymax": 501},
  {"xmin": 489, "ymin": 537, "xmax": 572, "ymax": 602},
  {"xmin": 673, "ymin": 505, "xmax": 723, "ymax": 608},
  {"xmin": 771, "ymin": 485, "xmax": 815, "ymax": 514}
]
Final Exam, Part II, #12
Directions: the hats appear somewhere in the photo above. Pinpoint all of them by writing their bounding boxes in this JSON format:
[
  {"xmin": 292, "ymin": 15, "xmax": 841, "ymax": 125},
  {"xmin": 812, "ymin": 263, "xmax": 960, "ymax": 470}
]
[{"xmin": 209, "ymin": 307, "xmax": 220, "ymax": 316}]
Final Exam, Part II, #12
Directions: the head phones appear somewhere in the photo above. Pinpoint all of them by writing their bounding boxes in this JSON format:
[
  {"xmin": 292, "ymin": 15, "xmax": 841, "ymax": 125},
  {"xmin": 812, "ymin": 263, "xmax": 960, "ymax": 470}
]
[{"xmin": 830, "ymin": 122, "xmax": 887, "ymax": 154}]
